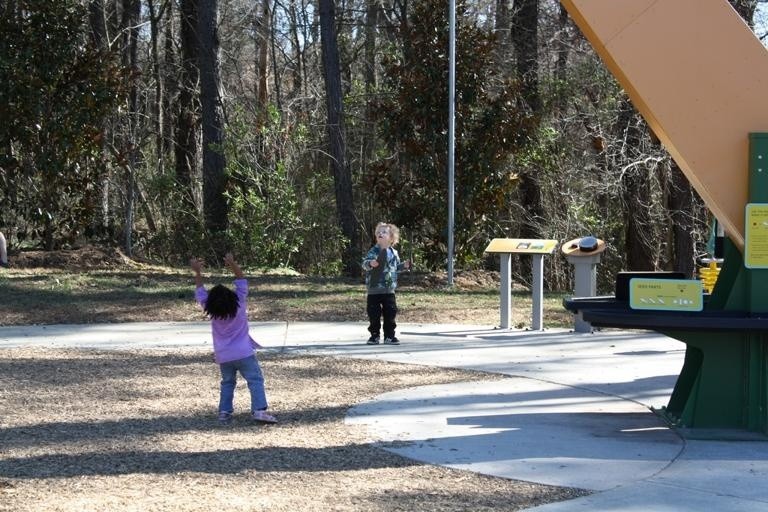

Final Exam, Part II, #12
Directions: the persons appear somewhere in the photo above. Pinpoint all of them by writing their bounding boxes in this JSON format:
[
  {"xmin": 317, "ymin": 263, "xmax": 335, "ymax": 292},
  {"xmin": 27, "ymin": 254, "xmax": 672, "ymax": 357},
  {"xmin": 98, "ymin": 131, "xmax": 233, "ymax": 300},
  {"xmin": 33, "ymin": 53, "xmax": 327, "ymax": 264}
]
[
  {"xmin": 189, "ymin": 252, "xmax": 278, "ymax": 424},
  {"xmin": 361, "ymin": 221, "xmax": 411, "ymax": 344}
]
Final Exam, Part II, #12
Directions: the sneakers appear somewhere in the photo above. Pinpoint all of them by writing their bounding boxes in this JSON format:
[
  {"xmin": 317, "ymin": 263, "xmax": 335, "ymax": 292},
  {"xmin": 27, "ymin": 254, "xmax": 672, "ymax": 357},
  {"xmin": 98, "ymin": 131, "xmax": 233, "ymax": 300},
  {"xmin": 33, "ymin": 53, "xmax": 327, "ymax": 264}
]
[
  {"xmin": 384, "ymin": 337, "xmax": 400, "ymax": 345},
  {"xmin": 218, "ymin": 411, "xmax": 232, "ymax": 421},
  {"xmin": 252, "ymin": 410, "xmax": 278, "ymax": 423},
  {"xmin": 367, "ymin": 335, "xmax": 380, "ymax": 344}
]
